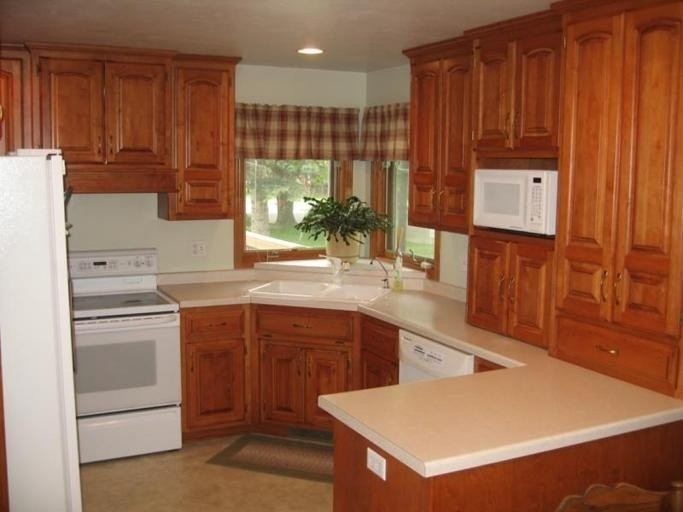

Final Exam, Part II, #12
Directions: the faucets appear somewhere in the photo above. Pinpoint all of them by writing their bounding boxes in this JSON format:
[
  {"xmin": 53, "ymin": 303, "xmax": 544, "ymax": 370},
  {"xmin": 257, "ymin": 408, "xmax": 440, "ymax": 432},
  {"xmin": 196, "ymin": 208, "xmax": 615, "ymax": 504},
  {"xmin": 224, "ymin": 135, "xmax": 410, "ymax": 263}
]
[
  {"xmin": 318, "ymin": 253, "xmax": 342, "ymax": 287},
  {"xmin": 369, "ymin": 257, "xmax": 390, "ymax": 289}
]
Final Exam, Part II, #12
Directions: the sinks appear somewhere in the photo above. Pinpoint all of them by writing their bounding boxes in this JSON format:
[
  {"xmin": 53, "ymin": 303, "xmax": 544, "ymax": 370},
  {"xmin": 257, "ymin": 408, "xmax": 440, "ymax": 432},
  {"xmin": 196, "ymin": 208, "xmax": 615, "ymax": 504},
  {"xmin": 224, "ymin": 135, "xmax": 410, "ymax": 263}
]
[
  {"xmin": 320, "ymin": 284, "xmax": 388, "ymax": 312},
  {"xmin": 249, "ymin": 280, "xmax": 329, "ymax": 308}
]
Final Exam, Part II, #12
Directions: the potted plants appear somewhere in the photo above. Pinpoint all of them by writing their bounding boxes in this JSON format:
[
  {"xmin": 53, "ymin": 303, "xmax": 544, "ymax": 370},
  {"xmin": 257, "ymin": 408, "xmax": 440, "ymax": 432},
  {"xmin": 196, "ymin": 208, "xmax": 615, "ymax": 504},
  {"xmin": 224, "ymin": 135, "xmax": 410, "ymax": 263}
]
[{"xmin": 295, "ymin": 195, "xmax": 393, "ymax": 259}]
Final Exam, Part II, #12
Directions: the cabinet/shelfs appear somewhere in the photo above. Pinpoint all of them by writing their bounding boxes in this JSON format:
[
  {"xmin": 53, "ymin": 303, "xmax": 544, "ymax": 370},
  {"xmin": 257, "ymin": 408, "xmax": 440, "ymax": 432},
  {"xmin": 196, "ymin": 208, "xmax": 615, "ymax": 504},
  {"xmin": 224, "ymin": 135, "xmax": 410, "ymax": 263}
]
[
  {"xmin": 0, "ymin": 43, "xmax": 34, "ymax": 156},
  {"xmin": 463, "ymin": 9, "xmax": 561, "ymax": 159},
  {"xmin": 550, "ymin": 0, "xmax": 683, "ymax": 335},
  {"xmin": 401, "ymin": 33, "xmax": 476, "ymax": 233},
  {"xmin": 159, "ymin": 52, "xmax": 243, "ymax": 220},
  {"xmin": 253, "ymin": 301, "xmax": 361, "ymax": 434},
  {"xmin": 465, "ymin": 227, "xmax": 556, "ymax": 348},
  {"xmin": 475, "ymin": 356, "xmax": 505, "ymax": 371},
  {"xmin": 179, "ymin": 303, "xmax": 255, "ymax": 440},
  {"xmin": 357, "ymin": 311, "xmax": 398, "ymax": 391},
  {"xmin": 547, "ymin": 311, "xmax": 683, "ymax": 399},
  {"xmin": 24, "ymin": 40, "xmax": 179, "ymax": 192}
]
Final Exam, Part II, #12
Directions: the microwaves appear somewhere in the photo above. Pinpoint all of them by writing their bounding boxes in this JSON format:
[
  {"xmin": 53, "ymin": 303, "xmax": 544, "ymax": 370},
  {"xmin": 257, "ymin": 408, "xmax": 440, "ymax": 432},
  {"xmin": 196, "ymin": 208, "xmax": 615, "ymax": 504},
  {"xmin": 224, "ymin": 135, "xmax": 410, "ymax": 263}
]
[{"xmin": 472, "ymin": 167, "xmax": 559, "ymax": 237}]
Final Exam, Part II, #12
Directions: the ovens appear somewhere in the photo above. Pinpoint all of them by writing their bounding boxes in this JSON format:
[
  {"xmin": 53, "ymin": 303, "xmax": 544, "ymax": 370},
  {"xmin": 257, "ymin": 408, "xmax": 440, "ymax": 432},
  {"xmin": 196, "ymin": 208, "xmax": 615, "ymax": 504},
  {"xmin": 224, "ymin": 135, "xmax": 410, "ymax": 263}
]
[{"xmin": 72, "ymin": 311, "xmax": 182, "ymax": 418}]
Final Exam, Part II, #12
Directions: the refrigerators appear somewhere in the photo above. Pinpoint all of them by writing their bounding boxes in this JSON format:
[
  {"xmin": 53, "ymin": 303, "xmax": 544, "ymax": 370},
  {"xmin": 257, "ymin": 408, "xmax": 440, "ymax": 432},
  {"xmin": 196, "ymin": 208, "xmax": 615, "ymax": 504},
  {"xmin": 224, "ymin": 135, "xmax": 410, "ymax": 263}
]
[{"xmin": 0, "ymin": 145, "xmax": 85, "ymax": 511}]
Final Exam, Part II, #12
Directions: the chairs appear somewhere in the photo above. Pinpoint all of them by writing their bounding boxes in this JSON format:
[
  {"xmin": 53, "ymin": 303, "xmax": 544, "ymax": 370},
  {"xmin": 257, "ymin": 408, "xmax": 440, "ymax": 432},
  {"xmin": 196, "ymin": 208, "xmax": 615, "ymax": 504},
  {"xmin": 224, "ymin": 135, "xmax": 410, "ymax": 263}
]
[{"xmin": 557, "ymin": 482, "xmax": 683, "ymax": 511}]
